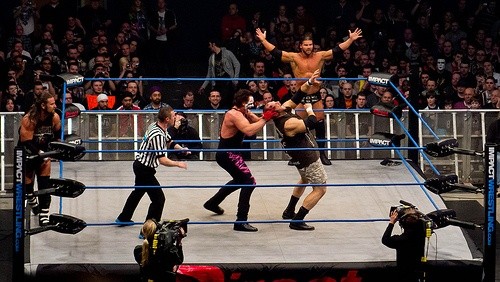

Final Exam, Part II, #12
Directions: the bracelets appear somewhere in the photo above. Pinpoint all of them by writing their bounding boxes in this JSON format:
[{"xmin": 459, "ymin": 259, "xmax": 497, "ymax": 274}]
[
  {"xmin": 166, "ymin": 27, "xmax": 170, "ymax": 32},
  {"xmin": 307, "ymin": 78, "xmax": 313, "ymax": 86},
  {"xmin": 306, "ymin": 107, "xmax": 312, "ymax": 113}
]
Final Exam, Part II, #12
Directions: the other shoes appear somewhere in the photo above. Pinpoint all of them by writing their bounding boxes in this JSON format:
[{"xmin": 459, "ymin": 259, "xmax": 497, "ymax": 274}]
[
  {"xmin": 281, "ymin": 211, "xmax": 297, "ymax": 220},
  {"xmin": 38, "ymin": 207, "xmax": 49, "ymax": 226},
  {"xmin": 290, "ymin": 218, "xmax": 315, "ymax": 231},
  {"xmin": 115, "ymin": 217, "xmax": 134, "ymax": 225},
  {"xmin": 320, "ymin": 151, "xmax": 331, "ymax": 165},
  {"xmin": 202, "ymin": 202, "xmax": 224, "ymax": 214},
  {"xmin": 233, "ymin": 223, "xmax": 258, "ymax": 232},
  {"xmin": 28, "ymin": 196, "xmax": 38, "ymax": 214}
]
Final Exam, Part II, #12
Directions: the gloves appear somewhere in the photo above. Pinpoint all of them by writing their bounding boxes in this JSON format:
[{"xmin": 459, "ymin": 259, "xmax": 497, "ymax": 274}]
[{"xmin": 262, "ymin": 105, "xmax": 277, "ymax": 122}]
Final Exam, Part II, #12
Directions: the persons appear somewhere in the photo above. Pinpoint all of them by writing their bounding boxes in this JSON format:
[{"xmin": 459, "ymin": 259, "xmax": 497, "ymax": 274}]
[
  {"xmin": 266, "ymin": 68, "xmax": 327, "ymax": 230},
  {"xmin": 204, "ymin": 89, "xmax": 278, "ymax": 232},
  {"xmin": 0, "ymin": 0, "xmax": 500, "ymax": 159},
  {"xmin": 134, "ymin": 219, "xmax": 184, "ymax": 282},
  {"xmin": 115, "ymin": 107, "xmax": 191, "ymax": 240},
  {"xmin": 18, "ymin": 91, "xmax": 62, "ymax": 227},
  {"xmin": 256, "ymin": 27, "xmax": 362, "ymax": 166},
  {"xmin": 381, "ymin": 208, "xmax": 425, "ymax": 282}
]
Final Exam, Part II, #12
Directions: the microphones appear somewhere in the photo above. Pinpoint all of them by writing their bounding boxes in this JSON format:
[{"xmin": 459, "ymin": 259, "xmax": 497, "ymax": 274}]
[
  {"xmin": 400, "ymin": 200, "xmax": 417, "ymax": 209},
  {"xmin": 178, "ymin": 218, "xmax": 190, "ymax": 227}
]
